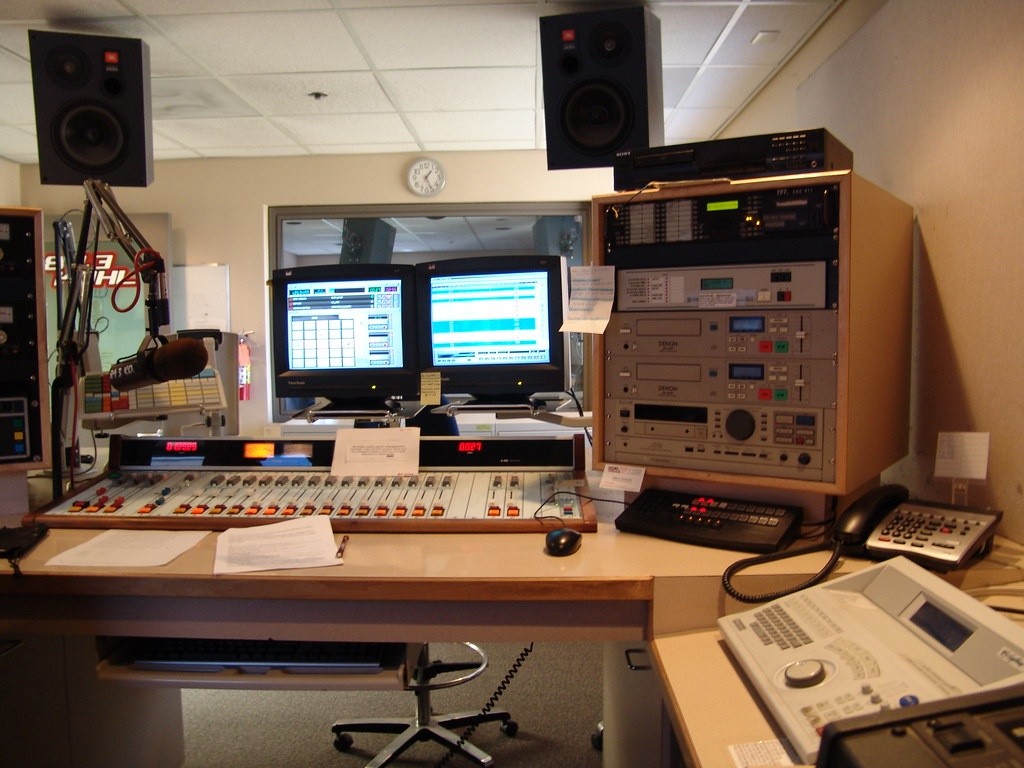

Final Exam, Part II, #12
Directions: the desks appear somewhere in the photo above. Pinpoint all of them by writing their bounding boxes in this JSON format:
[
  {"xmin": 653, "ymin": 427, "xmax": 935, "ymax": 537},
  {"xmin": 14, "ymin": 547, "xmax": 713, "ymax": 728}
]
[{"xmin": 0, "ymin": 474, "xmax": 1024, "ymax": 768}]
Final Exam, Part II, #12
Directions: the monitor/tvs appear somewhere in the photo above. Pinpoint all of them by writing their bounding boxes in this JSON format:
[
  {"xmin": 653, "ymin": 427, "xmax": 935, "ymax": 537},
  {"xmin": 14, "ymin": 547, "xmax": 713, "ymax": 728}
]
[
  {"xmin": 413, "ymin": 255, "xmax": 564, "ymax": 407},
  {"xmin": 270, "ymin": 263, "xmax": 417, "ymax": 417}
]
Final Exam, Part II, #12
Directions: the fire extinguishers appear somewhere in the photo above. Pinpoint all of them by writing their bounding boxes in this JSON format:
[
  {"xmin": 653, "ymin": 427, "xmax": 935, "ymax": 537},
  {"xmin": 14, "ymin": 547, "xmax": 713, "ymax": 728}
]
[{"xmin": 238, "ymin": 330, "xmax": 257, "ymax": 401}]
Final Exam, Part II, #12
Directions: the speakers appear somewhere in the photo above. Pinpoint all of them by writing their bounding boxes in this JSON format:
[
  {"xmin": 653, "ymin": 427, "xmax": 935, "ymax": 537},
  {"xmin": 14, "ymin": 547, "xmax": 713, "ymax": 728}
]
[
  {"xmin": 537, "ymin": 6, "xmax": 664, "ymax": 170},
  {"xmin": 530, "ymin": 216, "xmax": 583, "ymax": 268},
  {"xmin": 26, "ymin": 27, "xmax": 155, "ymax": 189},
  {"xmin": 339, "ymin": 217, "xmax": 396, "ymax": 265}
]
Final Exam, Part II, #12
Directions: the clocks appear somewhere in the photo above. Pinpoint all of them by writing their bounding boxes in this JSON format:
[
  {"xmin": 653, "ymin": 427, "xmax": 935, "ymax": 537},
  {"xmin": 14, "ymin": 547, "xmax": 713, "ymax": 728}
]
[{"xmin": 407, "ymin": 157, "xmax": 445, "ymax": 198}]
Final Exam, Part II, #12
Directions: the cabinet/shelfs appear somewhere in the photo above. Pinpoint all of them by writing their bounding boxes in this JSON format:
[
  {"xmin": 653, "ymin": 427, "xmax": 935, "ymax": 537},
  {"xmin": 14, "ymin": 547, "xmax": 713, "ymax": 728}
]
[{"xmin": 592, "ymin": 169, "xmax": 914, "ymax": 547}]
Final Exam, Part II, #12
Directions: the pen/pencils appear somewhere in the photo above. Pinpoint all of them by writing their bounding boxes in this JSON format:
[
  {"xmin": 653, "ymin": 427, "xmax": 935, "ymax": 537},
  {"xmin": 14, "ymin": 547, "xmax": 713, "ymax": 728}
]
[{"xmin": 336, "ymin": 534, "xmax": 350, "ymax": 558}]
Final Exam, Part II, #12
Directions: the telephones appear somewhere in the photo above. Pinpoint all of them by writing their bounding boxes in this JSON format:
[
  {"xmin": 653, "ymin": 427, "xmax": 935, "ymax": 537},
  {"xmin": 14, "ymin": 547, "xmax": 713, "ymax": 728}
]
[{"xmin": 833, "ymin": 484, "xmax": 1004, "ymax": 572}]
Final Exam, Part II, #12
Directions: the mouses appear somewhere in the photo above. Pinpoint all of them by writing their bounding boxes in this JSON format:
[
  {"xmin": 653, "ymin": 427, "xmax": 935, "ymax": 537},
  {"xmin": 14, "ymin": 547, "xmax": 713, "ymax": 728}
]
[{"xmin": 545, "ymin": 526, "xmax": 583, "ymax": 555}]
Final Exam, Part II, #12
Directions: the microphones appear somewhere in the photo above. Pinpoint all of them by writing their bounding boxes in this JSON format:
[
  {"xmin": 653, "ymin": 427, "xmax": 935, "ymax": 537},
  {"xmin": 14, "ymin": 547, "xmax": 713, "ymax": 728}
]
[{"xmin": 110, "ymin": 338, "xmax": 209, "ymax": 393}]
